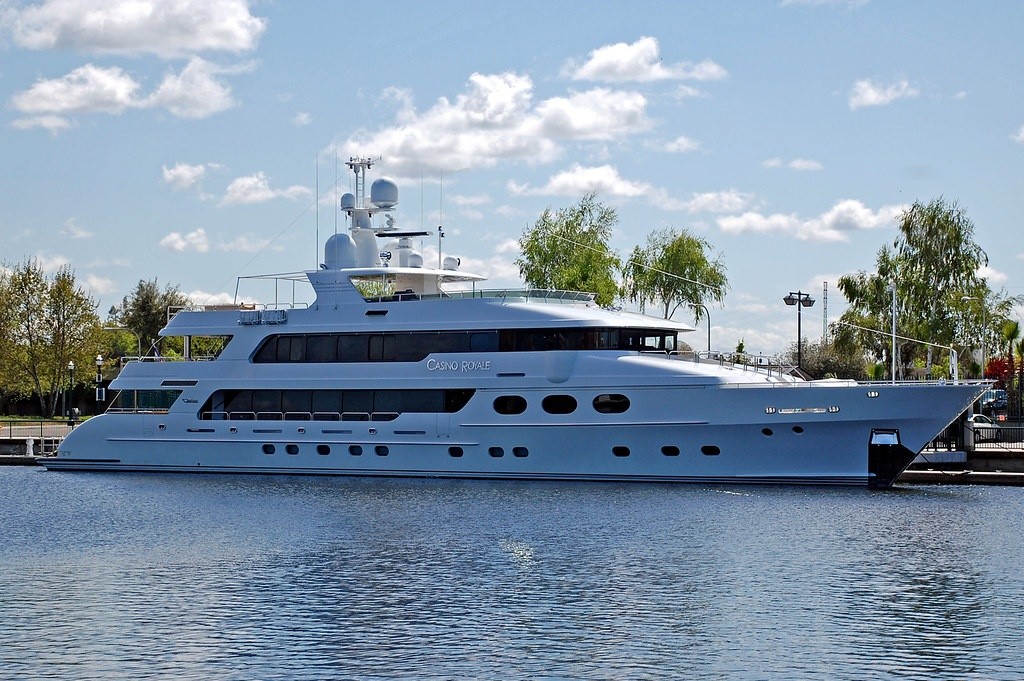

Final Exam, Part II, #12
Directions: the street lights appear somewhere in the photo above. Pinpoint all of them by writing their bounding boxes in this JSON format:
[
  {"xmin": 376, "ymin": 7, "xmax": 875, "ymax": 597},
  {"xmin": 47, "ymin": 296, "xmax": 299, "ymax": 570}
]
[
  {"xmin": 96, "ymin": 352, "xmax": 103, "ymax": 418},
  {"xmin": 687, "ymin": 303, "xmax": 710, "ymax": 361},
  {"xmin": 67, "ymin": 359, "xmax": 78, "ymax": 428},
  {"xmin": 784, "ymin": 291, "xmax": 817, "ymax": 367},
  {"xmin": 103, "ymin": 327, "xmax": 142, "ymax": 414},
  {"xmin": 960, "ymin": 294, "xmax": 985, "ymax": 381}
]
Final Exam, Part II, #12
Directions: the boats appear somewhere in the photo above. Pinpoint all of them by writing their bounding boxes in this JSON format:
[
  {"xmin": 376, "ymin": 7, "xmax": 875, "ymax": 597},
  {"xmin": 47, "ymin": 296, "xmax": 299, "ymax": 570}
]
[{"xmin": 37, "ymin": 157, "xmax": 1000, "ymax": 483}]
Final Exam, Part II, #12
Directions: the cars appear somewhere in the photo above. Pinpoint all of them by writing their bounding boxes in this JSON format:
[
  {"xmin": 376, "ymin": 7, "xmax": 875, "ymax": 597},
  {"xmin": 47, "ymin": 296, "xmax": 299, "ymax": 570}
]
[
  {"xmin": 976, "ymin": 390, "xmax": 1009, "ymax": 413},
  {"xmin": 972, "ymin": 414, "xmax": 1003, "ymax": 443}
]
